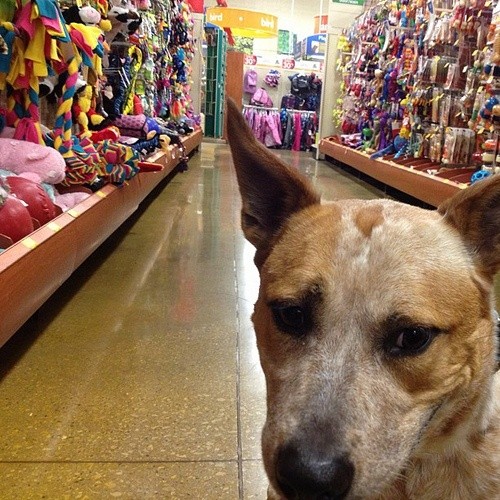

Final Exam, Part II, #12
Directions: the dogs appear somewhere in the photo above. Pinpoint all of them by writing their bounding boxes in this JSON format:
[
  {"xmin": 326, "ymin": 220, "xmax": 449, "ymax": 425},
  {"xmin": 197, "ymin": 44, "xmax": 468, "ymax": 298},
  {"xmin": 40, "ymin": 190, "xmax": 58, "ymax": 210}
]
[{"xmin": 224, "ymin": 95, "xmax": 499, "ymax": 500}]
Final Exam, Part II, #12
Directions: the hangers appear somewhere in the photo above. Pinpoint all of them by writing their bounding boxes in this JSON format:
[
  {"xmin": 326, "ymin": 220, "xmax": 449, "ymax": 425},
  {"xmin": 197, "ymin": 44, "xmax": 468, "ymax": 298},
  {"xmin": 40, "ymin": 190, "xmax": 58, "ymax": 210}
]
[{"xmin": 243, "ymin": 105, "xmax": 315, "ymax": 119}]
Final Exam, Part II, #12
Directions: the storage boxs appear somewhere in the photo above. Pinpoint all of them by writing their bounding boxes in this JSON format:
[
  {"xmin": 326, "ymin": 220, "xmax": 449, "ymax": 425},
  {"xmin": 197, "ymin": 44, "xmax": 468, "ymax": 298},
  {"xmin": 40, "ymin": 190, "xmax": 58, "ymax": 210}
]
[{"xmin": 300, "ymin": 32, "xmax": 326, "ymax": 56}]
